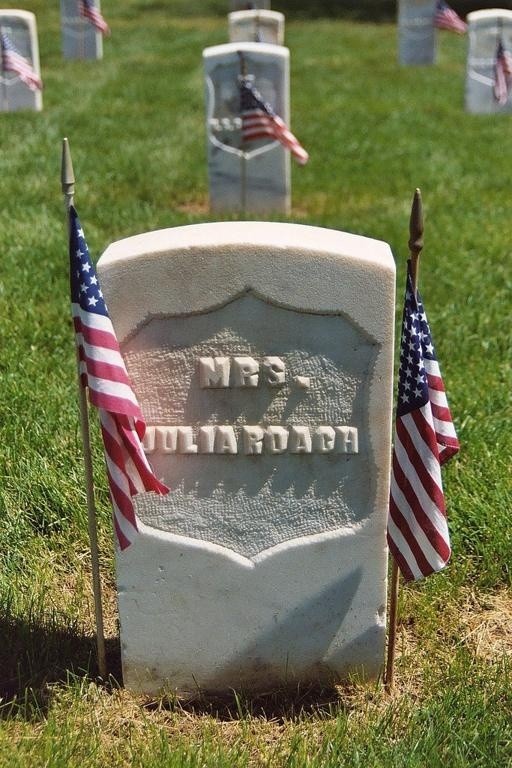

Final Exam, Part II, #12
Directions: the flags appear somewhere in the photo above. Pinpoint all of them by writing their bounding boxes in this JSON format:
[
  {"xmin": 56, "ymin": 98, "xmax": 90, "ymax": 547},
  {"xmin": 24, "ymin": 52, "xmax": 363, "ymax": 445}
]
[
  {"xmin": 386, "ymin": 260, "xmax": 459, "ymax": 586},
  {"xmin": 237, "ymin": 73, "xmax": 309, "ymax": 167},
  {"xmin": 433, "ymin": 0, "xmax": 468, "ymax": 35},
  {"xmin": 0, "ymin": 30, "xmax": 44, "ymax": 92},
  {"xmin": 491, "ymin": 37, "xmax": 512, "ymax": 108},
  {"xmin": 68, "ymin": 202, "xmax": 170, "ymax": 552},
  {"xmin": 79, "ymin": 0, "xmax": 111, "ymax": 37}
]
